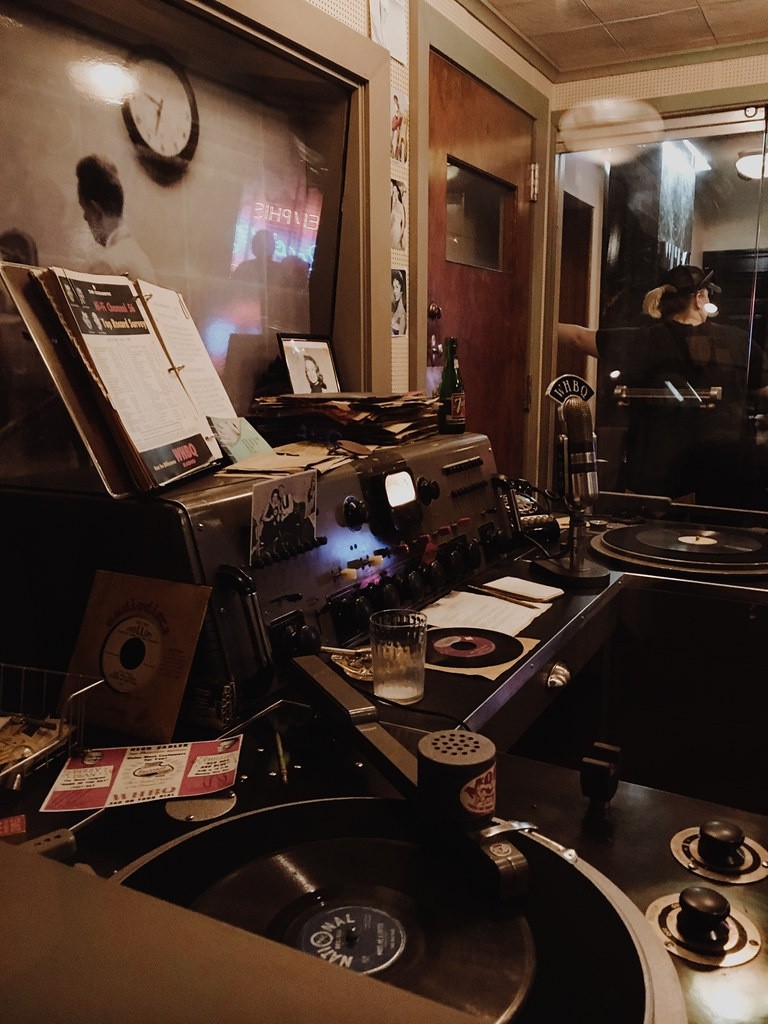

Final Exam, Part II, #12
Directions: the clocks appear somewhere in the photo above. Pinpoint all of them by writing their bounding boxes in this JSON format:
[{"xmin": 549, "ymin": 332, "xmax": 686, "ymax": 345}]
[{"xmin": 122, "ymin": 44, "xmax": 200, "ymax": 176}]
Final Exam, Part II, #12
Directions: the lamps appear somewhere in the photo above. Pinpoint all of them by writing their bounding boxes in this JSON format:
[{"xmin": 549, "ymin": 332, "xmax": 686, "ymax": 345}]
[{"xmin": 735, "ymin": 149, "xmax": 768, "ymax": 180}]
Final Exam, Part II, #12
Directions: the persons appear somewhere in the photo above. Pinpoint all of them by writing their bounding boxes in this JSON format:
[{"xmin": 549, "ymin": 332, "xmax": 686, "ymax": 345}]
[
  {"xmin": 556, "ymin": 264, "xmax": 766, "ymax": 500},
  {"xmin": 390, "ymin": 181, "xmax": 407, "ymax": 251},
  {"xmin": 304, "ymin": 354, "xmax": 327, "ymax": 393},
  {"xmin": 391, "ymin": 269, "xmax": 406, "ymax": 335},
  {"xmin": 258, "ymin": 485, "xmax": 301, "ymax": 553},
  {"xmin": 1, "ymin": 154, "xmax": 281, "ymax": 331},
  {"xmin": 392, "ymin": 95, "xmax": 403, "ymax": 161}
]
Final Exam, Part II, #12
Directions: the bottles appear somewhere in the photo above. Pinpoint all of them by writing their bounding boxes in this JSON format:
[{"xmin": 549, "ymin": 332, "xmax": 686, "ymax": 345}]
[{"xmin": 437, "ymin": 336, "xmax": 466, "ymax": 434}]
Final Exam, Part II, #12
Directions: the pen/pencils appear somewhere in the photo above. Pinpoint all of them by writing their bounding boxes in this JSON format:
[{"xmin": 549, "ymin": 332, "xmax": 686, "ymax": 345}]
[{"xmin": 320, "ymin": 645, "xmax": 362, "ymax": 658}]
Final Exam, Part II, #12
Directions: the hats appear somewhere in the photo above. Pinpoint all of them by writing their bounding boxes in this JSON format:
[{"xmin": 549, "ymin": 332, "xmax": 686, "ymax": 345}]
[{"xmin": 661, "ymin": 264, "xmax": 715, "ymax": 299}]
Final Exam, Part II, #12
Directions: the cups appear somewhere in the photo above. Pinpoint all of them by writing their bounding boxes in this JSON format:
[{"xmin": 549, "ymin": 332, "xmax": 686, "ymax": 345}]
[{"xmin": 368, "ymin": 608, "xmax": 427, "ymax": 705}]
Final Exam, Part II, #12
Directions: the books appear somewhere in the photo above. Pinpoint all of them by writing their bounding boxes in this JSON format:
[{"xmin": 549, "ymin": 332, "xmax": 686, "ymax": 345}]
[{"xmin": 23, "ymin": 266, "xmax": 240, "ymax": 491}]
